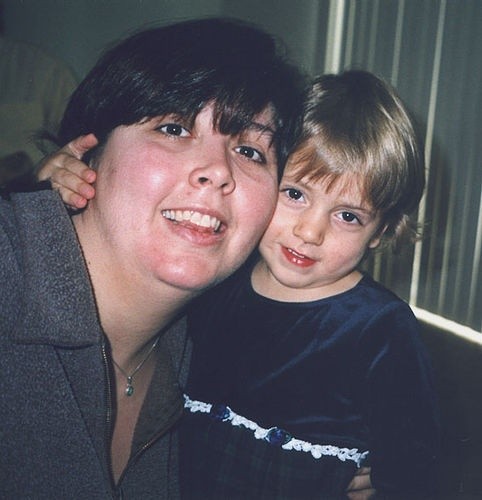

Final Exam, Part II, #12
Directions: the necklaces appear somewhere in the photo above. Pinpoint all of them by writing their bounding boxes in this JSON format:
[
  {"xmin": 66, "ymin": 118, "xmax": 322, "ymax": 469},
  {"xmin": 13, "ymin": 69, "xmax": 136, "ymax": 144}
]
[{"xmin": 108, "ymin": 333, "xmax": 161, "ymax": 397}]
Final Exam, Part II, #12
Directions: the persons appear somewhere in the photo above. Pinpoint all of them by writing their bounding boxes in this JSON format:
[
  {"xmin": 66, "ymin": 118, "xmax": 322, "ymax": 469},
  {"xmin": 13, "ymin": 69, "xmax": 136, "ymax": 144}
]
[
  {"xmin": 26, "ymin": 61, "xmax": 439, "ymax": 500},
  {"xmin": 0, "ymin": 14, "xmax": 378, "ymax": 500}
]
